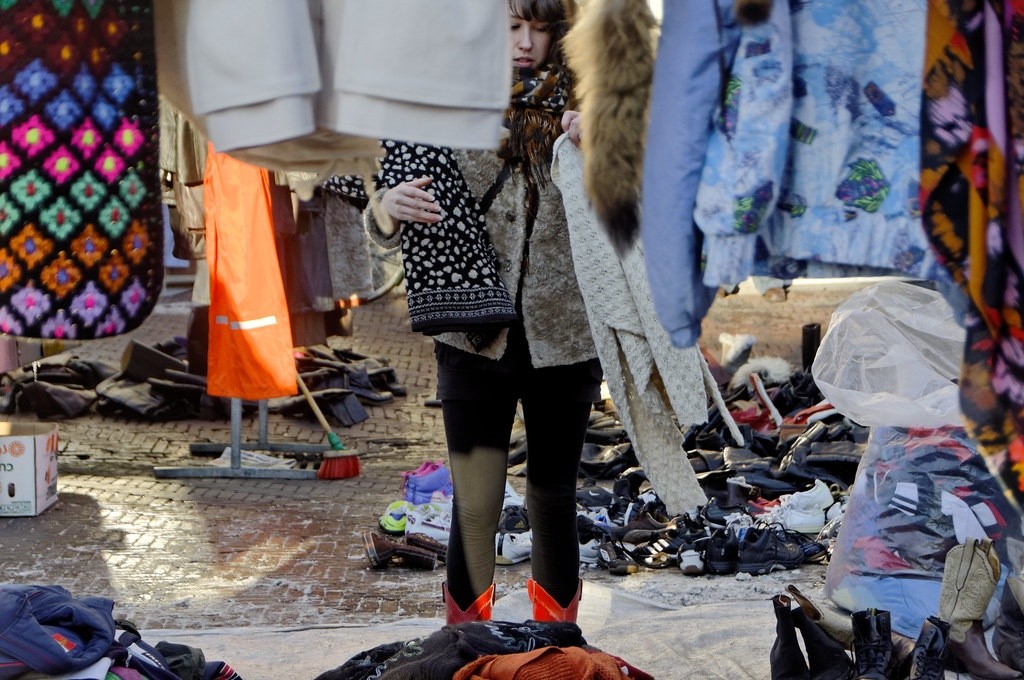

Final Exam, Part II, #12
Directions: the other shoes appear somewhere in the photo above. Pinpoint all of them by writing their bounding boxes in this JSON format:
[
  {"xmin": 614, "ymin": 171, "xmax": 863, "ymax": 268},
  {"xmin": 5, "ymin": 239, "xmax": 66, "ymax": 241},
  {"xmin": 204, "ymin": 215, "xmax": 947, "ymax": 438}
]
[{"xmin": 362, "ymin": 323, "xmax": 870, "ymax": 576}]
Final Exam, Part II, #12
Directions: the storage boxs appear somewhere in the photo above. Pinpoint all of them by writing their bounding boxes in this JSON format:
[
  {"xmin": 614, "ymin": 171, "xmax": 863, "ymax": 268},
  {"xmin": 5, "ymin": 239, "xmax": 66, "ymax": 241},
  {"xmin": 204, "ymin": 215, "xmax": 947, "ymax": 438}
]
[{"xmin": 0, "ymin": 421, "xmax": 59, "ymax": 515}]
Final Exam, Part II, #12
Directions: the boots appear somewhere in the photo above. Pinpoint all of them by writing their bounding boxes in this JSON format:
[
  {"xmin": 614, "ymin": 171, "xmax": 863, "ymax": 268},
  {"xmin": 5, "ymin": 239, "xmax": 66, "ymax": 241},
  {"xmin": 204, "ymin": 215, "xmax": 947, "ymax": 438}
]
[
  {"xmin": 442, "ymin": 579, "xmax": 496, "ymax": 626},
  {"xmin": 770, "ymin": 584, "xmax": 952, "ymax": 679},
  {"xmin": 528, "ymin": 577, "xmax": 583, "ymax": 624},
  {"xmin": 938, "ymin": 537, "xmax": 1023, "ymax": 680},
  {"xmin": 992, "ymin": 574, "xmax": 1023, "ymax": 673},
  {"xmin": 0, "ymin": 336, "xmax": 408, "ymax": 428}
]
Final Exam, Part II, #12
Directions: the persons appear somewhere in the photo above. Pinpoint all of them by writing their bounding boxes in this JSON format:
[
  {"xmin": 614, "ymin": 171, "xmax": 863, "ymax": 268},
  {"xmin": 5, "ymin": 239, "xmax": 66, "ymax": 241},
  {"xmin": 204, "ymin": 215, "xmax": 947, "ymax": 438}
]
[{"xmin": 362, "ymin": 0, "xmax": 585, "ymax": 625}]
